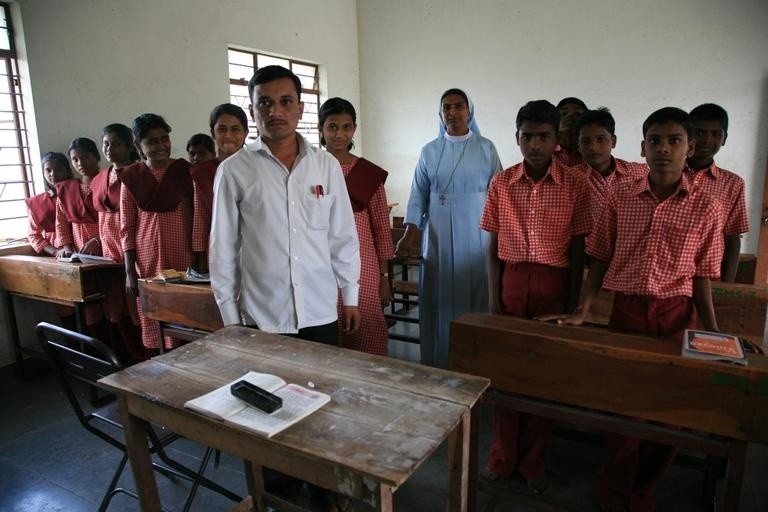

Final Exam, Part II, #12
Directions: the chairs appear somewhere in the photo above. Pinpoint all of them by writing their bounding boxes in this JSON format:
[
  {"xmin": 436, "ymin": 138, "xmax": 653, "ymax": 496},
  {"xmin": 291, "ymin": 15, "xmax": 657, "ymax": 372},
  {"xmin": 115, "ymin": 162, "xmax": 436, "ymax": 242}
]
[{"xmin": 36, "ymin": 322, "xmax": 244, "ymax": 512}]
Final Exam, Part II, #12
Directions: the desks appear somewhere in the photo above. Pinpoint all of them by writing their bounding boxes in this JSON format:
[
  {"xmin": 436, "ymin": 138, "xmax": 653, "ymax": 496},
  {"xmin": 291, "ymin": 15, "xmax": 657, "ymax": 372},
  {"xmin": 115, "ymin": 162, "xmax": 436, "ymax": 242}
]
[
  {"xmin": 0, "ymin": 255, "xmax": 131, "ymax": 406},
  {"xmin": 449, "ymin": 313, "xmax": 767, "ymax": 511},
  {"xmin": 138, "ymin": 274, "xmax": 224, "ymax": 357},
  {"xmin": 97, "ymin": 326, "xmax": 491, "ymax": 512}
]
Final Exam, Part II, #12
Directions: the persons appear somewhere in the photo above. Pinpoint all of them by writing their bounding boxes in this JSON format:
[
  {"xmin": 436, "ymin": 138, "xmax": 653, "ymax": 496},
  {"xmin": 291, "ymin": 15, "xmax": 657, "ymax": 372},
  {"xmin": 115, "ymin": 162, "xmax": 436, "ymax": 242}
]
[
  {"xmin": 89, "ymin": 124, "xmax": 148, "ymax": 366},
  {"xmin": 185, "ymin": 132, "xmax": 217, "ymax": 168},
  {"xmin": 569, "ymin": 108, "xmax": 651, "ymax": 265},
  {"xmin": 535, "ymin": 105, "xmax": 767, "ymax": 512},
  {"xmin": 21, "ymin": 151, "xmax": 79, "ymax": 355},
  {"xmin": 53, "ymin": 137, "xmax": 101, "ymax": 367},
  {"xmin": 681, "ymin": 102, "xmax": 751, "ymax": 285},
  {"xmin": 314, "ymin": 96, "xmax": 397, "ymax": 358},
  {"xmin": 552, "ymin": 97, "xmax": 589, "ymax": 166},
  {"xmin": 208, "ymin": 65, "xmax": 362, "ymax": 512},
  {"xmin": 117, "ymin": 113, "xmax": 194, "ymax": 358},
  {"xmin": 481, "ymin": 100, "xmax": 596, "ymax": 501},
  {"xmin": 394, "ymin": 87, "xmax": 507, "ymax": 441},
  {"xmin": 192, "ymin": 102, "xmax": 249, "ymax": 273}
]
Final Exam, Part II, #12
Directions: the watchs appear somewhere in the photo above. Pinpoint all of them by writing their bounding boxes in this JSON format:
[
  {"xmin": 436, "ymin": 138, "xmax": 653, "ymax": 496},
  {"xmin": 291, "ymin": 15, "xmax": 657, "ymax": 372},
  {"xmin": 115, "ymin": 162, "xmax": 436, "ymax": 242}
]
[{"xmin": 380, "ymin": 272, "xmax": 389, "ymax": 278}]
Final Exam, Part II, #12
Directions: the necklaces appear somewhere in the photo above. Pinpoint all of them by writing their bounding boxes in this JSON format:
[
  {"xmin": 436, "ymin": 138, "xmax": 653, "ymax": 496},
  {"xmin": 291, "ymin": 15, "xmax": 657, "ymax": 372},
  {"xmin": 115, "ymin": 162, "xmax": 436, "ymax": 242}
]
[{"xmin": 435, "ymin": 138, "xmax": 468, "ymax": 205}]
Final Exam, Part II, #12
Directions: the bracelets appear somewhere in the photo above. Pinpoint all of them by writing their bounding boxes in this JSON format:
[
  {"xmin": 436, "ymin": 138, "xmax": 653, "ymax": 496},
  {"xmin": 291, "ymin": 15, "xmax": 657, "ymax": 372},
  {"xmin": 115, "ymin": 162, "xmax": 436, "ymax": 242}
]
[{"xmin": 91, "ymin": 237, "xmax": 100, "ymax": 247}]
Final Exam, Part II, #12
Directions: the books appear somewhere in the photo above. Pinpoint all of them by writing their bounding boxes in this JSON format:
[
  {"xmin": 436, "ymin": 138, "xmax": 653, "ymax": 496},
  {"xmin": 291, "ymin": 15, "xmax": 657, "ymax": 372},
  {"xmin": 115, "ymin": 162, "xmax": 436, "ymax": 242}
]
[
  {"xmin": 57, "ymin": 253, "xmax": 114, "ymax": 265},
  {"xmin": 159, "ymin": 269, "xmax": 181, "ymax": 282},
  {"xmin": 152, "ymin": 272, "xmax": 187, "ymax": 283},
  {"xmin": 682, "ymin": 329, "xmax": 749, "ymax": 366},
  {"xmin": 183, "ymin": 370, "xmax": 331, "ymax": 439}
]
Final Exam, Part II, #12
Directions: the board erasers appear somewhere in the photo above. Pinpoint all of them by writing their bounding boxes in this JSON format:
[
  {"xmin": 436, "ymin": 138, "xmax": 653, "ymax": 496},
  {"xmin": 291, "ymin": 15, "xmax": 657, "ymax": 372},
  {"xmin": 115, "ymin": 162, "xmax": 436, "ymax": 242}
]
[{"xmin": 229, "ymin": 379, "xmax": 284, "ymax": 415}]
